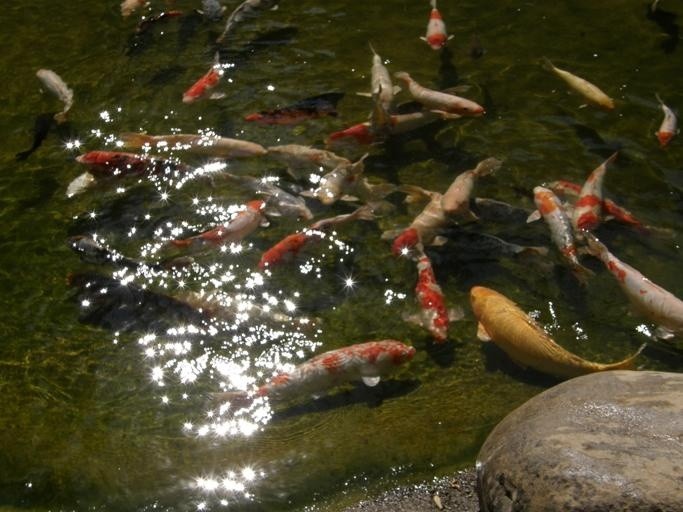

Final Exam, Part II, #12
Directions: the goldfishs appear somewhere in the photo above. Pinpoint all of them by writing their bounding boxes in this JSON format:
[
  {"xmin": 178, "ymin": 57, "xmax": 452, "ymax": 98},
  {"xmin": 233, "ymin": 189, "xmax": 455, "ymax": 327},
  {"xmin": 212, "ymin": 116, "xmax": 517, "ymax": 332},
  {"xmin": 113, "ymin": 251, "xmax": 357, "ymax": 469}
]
[{"xmin": 35, "ymin": 0, "xmax": 683, "ymax": 344}]
